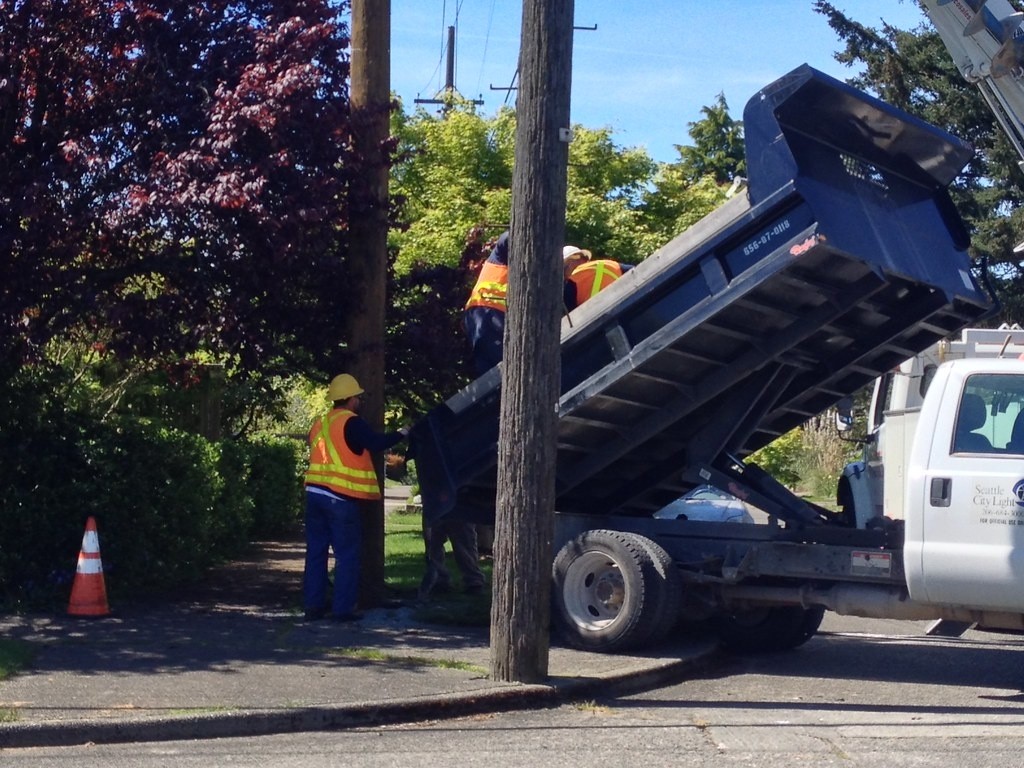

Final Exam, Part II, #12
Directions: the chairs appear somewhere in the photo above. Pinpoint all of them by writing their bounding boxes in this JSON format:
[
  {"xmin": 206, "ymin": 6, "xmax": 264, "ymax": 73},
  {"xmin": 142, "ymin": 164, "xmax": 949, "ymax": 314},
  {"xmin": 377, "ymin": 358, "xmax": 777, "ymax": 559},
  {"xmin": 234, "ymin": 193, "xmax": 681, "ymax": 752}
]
[
  {"xmin": 1006, "ymin": 408, "xmax": 1024, "ymax": 454},
  {"xmin": 954, "ymin": 393, "xmax": 997, "ymax": 453}
]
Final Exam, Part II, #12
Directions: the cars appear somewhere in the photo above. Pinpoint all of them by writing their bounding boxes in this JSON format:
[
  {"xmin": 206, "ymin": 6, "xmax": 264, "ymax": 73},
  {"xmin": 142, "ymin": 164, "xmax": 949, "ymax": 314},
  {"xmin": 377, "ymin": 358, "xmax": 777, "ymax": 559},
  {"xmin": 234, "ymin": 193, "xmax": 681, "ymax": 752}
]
[{"xmin": 653, "ymin": 481, "xmax": 788, "ymax": 524}]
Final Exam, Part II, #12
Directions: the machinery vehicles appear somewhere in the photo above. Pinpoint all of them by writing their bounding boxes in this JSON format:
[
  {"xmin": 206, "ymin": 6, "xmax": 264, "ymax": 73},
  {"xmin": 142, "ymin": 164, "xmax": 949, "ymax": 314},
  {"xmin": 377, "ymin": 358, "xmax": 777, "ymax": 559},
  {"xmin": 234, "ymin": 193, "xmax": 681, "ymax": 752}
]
[{"xmin": 837, "ymin": 1, "xmax": 1024, "ymax": 636}]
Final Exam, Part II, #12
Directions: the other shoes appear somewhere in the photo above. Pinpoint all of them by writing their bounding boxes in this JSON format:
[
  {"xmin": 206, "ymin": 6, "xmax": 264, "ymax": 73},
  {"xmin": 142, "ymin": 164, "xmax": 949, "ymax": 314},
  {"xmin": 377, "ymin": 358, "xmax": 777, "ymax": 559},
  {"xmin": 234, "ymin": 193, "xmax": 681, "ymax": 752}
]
[
  {"xmin": 332, "ymin": 612, "xmax": 365, "ymax": 622},
  {"xmin": 304, "ymin": 610, "xmax": 327, "ymax": 621}
]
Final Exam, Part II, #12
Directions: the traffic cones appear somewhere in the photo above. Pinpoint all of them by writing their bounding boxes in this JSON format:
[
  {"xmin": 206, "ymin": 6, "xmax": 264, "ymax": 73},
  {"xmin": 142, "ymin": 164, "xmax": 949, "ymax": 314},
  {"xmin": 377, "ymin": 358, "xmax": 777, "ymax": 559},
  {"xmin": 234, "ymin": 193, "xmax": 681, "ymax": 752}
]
[{"xmin": 65, "ymin": 516, "xmax": 111, "ymax": 616}]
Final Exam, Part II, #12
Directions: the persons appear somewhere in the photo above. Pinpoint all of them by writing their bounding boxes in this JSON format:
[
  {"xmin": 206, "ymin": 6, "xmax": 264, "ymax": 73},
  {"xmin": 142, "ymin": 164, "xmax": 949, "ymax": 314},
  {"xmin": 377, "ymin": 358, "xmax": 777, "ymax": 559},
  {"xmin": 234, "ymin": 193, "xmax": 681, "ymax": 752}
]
[
  {"xmin": 563, "ymin": 246, "xmax": 638, "ymax": 316},
  {"xmin": 301, "ymin": 373, "xmax": 413, "ymax": 625},
  {"xmin": 460, "ymin": 229, "xmax": 510, "ymax": 382}
]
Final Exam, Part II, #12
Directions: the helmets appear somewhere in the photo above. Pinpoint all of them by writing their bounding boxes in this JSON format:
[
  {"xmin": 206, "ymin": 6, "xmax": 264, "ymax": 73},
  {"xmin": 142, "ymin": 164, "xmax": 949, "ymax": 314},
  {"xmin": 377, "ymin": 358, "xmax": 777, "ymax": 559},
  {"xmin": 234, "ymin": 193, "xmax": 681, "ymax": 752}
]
[
  {"xmin": 325, "ymin": 374, "xmax": 364, "ymax": 402},
  {"xmin": 563, "ymin": 246, "xmax": 591, "ymax": 263}
]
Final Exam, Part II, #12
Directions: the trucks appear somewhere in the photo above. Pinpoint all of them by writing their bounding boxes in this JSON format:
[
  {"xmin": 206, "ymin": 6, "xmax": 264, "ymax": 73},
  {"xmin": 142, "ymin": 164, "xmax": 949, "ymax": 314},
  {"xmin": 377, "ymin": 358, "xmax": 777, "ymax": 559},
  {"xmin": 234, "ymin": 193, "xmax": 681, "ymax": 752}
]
[{"xmin": 408, "ymin": 64, "xmax": 1024, "ymax": 653}]
